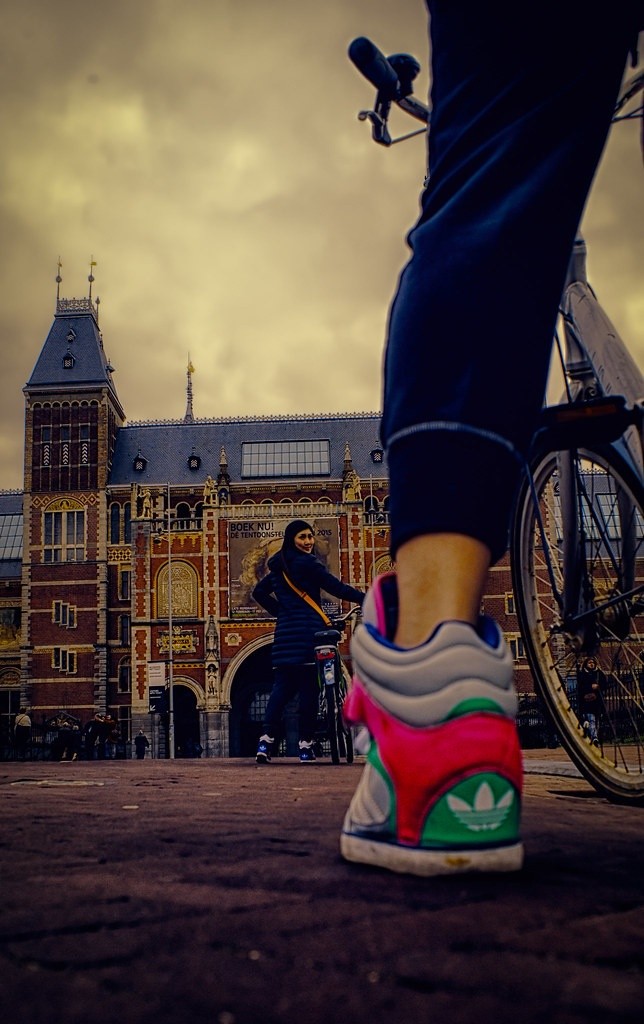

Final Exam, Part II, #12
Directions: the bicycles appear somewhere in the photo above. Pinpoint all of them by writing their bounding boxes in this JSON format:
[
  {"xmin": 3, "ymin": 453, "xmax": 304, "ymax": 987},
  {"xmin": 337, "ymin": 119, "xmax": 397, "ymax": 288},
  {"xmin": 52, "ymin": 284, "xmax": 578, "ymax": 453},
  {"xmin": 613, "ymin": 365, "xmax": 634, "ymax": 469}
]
[
  {"xmin": 313, "ymin": 605, "xmax": 363, "ymax": 766},
  {"xmin": 346, "ymin": 31, "xmax": 644, "ymax": 808}
]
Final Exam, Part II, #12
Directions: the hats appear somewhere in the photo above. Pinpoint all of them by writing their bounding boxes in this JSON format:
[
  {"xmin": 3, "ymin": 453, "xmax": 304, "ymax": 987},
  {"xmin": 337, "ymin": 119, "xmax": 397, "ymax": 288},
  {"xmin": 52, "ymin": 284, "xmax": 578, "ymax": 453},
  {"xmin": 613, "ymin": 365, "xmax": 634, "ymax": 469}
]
[
  {"xmin": 63, "ymin": 722, "xmax": 69, "ymax": 726},
  {"xmin": 138, "ymin": 730, "xmax": 143, "ymax": 734}
]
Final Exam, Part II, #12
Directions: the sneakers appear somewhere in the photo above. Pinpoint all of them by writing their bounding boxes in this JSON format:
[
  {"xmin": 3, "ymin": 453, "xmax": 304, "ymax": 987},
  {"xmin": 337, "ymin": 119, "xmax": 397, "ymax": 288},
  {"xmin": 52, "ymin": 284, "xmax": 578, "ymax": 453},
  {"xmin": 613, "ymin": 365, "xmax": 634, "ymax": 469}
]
[
  {"xmin": 255, "ymin": 734, "xmax": 274, "ymax": 764},
  {"xmin": 298, "ymin": 740, "xmax": 317, "ymax": 763},
  {"xmin": 337, "ymin": 570, "xmax": 526, "ymax": 875}
]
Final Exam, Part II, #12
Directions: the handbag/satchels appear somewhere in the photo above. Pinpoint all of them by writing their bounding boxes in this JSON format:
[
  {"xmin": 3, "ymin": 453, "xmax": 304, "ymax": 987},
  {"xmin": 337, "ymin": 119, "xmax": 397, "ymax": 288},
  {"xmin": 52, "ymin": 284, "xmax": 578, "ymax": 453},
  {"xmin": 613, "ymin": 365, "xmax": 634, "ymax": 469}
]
[{"xmin": 14, "ymin": 724, "xmax": 18, "ymax": 734}]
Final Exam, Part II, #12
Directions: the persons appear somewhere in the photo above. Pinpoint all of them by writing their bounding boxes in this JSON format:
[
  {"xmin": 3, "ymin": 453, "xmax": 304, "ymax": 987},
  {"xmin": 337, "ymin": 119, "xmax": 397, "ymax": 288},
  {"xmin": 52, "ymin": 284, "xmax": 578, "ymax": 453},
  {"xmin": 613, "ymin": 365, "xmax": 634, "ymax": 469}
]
[
  {"xmin": 339, "ymin": 0, "xmax": 644, "ymax": 872},
  {"xmin": 203, "ymin": 476, "xmax": 214, "ymax": 504},
  {"xmin": 14, "ymin": 708, "xmax": 32, "ymax": 761},
  {"xmin": 138, "ymin": 487, "xmax": 151, "ymax": 517},
  {"xmin": 577, "ymin": 658, "xmax": 608, "ymax": 748},
  {"xmin": 252, "ymin": 520, "xmax": 366, "ymax": 763},
  {"xmin": 54, "ymin": 713, "xmax": 150, "ymax": 760},
  {"xmin": 348, "ymin": 470, "xmax": 361, "ymax": 500},
  {"xmin": 207, "ymin": 667, "xmax": 217, "ymax": 695}
]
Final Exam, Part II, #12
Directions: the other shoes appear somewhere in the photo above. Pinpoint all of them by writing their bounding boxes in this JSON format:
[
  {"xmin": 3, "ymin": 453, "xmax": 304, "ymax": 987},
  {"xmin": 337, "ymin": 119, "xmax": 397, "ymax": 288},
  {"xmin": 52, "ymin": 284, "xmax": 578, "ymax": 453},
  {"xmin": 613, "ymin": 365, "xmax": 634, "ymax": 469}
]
[
  {"xmin": 71, "ymin": 753, "xmax": 77, "ymax": 760},
  {"xmin": 62, "ymin": 751, "xmax": 67, "ymax": 758}
]
